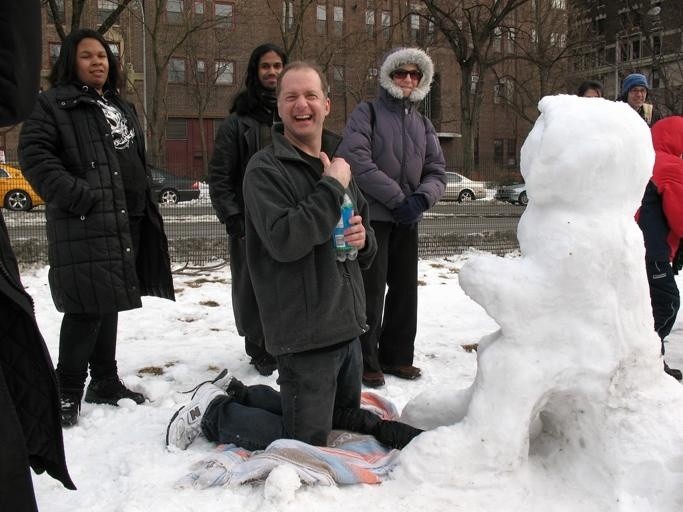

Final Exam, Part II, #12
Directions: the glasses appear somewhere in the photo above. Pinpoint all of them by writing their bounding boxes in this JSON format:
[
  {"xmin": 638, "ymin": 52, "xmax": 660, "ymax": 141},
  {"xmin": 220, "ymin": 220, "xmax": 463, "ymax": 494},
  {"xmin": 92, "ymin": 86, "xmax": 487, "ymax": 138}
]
[
  {"xmin": 391, "ymin": 69, "xmax": 422, "ymax": 81},
  {"xmin": 629, "ymin": 86, "xmax": 647, "ymax": 95}
]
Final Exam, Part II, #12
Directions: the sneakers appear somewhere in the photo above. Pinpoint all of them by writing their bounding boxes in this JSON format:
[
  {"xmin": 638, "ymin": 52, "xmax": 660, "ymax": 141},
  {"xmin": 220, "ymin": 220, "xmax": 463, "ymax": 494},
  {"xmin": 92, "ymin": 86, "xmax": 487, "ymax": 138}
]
[
  {"xmin": 212, "ymin": 367, "xmax": 233, "ymax": 392},
  {"xmin": 164, "ymin": 382, "xmax": 229, "ymax": 453}
]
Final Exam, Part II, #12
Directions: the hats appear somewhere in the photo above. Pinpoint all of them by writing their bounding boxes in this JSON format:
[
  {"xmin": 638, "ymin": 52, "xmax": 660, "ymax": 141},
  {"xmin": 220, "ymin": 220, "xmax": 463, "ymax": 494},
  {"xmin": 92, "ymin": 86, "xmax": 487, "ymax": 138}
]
[{"xmin": 623, "ymin": 73, "xmax": 649, "ymax": 95}]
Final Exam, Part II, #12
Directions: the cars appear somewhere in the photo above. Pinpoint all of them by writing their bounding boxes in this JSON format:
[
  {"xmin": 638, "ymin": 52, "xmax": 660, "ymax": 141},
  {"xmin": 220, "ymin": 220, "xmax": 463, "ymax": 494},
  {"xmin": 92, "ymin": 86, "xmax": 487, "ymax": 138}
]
[
  {"xmin": 440, "ymin": 168, "xmax": 488, "ymax": 203},
  {"xmin": 0, "ymin": 161, "xmax": 46, "ymax": 211},
  {"xmin": 496, "ymin": 182, "xmax": 530, "ymax": 205},
  {"xmin": 146, "ymin": 163, "xmax": 201, "ymax": 206}
]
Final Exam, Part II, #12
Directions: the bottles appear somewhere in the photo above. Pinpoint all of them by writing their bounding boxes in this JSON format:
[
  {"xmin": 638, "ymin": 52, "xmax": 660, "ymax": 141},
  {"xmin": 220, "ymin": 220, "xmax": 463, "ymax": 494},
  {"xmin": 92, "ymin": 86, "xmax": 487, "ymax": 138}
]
[{"xmin": 333, "ymin": 190, "xmax": 361, "ymax": 263}]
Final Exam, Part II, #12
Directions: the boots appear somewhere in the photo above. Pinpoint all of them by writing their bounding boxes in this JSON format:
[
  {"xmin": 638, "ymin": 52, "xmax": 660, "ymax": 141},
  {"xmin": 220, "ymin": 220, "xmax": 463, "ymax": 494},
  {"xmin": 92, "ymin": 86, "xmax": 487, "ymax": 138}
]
[
  {"xmin": 54, "ymin": 367, "xmax": 87, "ymax": 425},
  {"xmin": 86, "ymin": 360, "xmax": 146, "ymax": 406}
]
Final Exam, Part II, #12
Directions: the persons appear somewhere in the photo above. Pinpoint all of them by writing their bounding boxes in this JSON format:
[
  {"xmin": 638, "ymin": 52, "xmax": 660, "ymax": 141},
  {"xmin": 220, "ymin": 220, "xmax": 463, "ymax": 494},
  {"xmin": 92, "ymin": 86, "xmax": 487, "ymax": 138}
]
[
  {"xmin": 578, "ymin": 79, "xmax": 606, "ymax": 100},
  {"xmin": 634, "ymin": 114, "xmax": 683, "ymax": 380},
  {"xmin": 2, "ymin": 209, "xmax": 77, "ymax": 511},
  {"xmin": 339, "ymin": 44, "xmax": 449, "ymax": 390},
  {"xmin": 164, "ymin": 62, "xmax": 379, "ymax": 453},
  {"xmin": 206, "ymin": 43, "xmax": 290, "ymax": 376},
  {"xmin": 617, "ymin": 71, "xmax": 650, "ymax": 120},
  {"xmin": 16, "ymin": 26, "xmax": 177, "ymax": 428}
]
